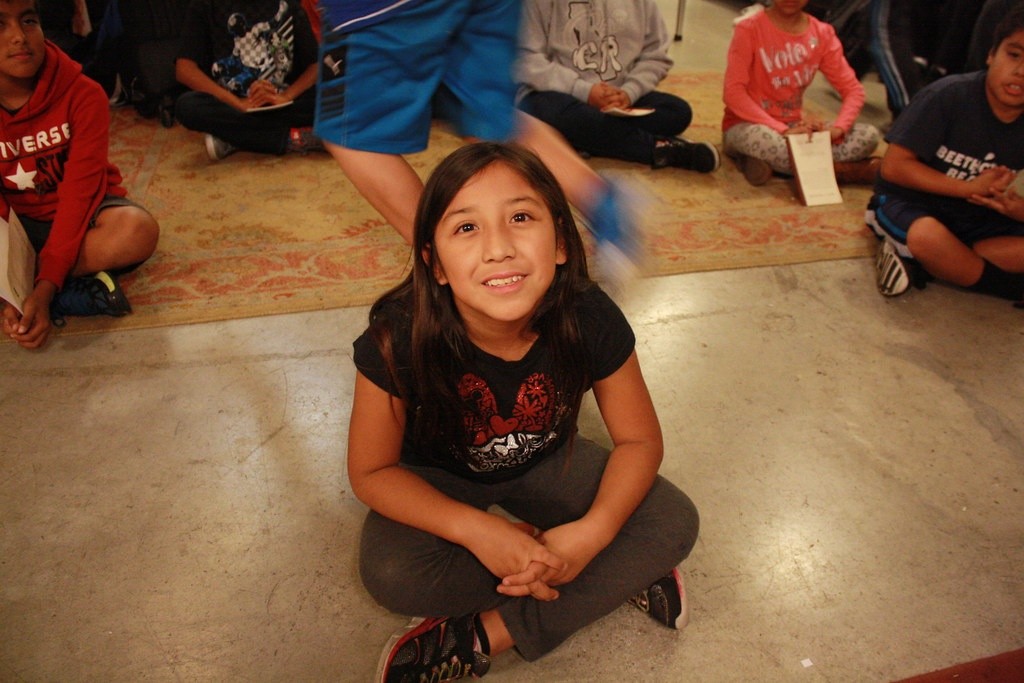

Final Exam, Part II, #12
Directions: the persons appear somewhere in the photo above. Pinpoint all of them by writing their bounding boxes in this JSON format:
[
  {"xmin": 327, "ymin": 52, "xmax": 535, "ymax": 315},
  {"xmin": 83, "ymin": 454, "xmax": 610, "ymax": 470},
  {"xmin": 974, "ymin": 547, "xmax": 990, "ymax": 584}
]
[
  {"xmin": 862, "ymin": 2, "xmax": 1024, "ymax": 309},
  {"xmin": 36, "ymin": 0, "xmax": 321, "ymax": 159},
  {"xmin": 834, "ymin": 0, "xmax": 919, "ymax": 124},
  {"xmin": 513, "ymin": 0, "xmax": 721, "ymax": 174},
  {"xmin": 0, "ymin": 0, "xmax": 161, "ymax": 349},
  {"xmin": 345, "ymin": 141, "xmax": 700, "ymax": 683},
  {"xmin": 719, "ymin": 1, "xmax": 883, "ymax": 187},
  {"xmin": 311, "ymin": 1, "xmax": 650, "ymax": 288}
]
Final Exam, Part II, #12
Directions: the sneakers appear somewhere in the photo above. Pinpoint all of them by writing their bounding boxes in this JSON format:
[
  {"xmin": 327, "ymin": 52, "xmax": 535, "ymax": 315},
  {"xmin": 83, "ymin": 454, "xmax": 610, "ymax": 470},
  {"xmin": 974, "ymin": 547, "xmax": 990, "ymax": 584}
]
[
  {"xmin": 377, "ymin": 616, "xmax": 490, "ymax": 683},
  {"xmin": 626, "ymin": 563, "xmax": 686, "ymax": 629},
  {"xmin": 49, "ymin": 270, "xmax": 130, "ymax": 327},
  {"xmin": 650, "ymin": 136, "xmax": 720, "ymax": 173}
]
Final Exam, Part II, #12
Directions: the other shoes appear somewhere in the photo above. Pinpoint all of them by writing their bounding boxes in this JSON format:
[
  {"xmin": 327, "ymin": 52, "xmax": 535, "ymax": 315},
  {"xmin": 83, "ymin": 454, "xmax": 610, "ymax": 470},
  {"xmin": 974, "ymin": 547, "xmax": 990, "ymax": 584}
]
[
  {"xmin": 875, "ymin": 237, "xmax": 926, "ymax": 296},
  {"xmin": 850, "ymin": 155, "xmax": 885, "ymax": 187},
  {"xmin": 288, "ymin": 125, "xmax": 325, "ymax": 156},
  {"xmin": 204, "ymin": 132, "xmax": 235, "ymax": 161},
  {"xmin": 596, "ymin": 168, "xmax": 657, "ymax": 295},
  {"xmin": 741, "ymin": 155, "xmax": 771, "ymax": 188}
]
